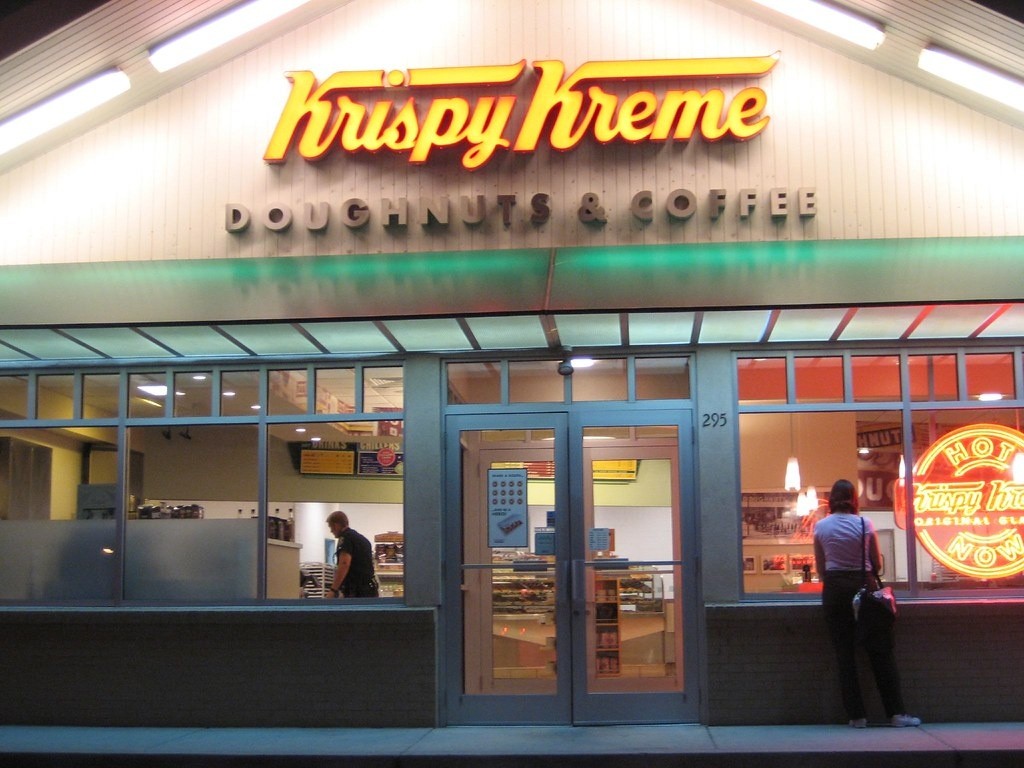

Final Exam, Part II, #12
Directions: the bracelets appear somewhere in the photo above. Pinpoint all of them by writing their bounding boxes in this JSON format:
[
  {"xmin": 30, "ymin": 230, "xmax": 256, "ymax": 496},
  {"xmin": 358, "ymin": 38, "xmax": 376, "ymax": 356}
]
[{"xmin": 330, "ymin": 588, "xmax": 337, "ymax": 593}]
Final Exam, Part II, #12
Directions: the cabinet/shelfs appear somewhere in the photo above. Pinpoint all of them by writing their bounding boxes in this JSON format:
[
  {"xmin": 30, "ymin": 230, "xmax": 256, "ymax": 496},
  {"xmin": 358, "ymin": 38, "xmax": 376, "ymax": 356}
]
[{"xmin": 373, "ymin": 543, "xmax": 655, "ymax": 611}]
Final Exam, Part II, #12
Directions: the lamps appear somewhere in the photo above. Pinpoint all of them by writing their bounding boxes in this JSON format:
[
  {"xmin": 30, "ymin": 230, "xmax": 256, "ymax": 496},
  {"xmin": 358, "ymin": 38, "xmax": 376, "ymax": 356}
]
[
  {"xmin": 784, "ymin": 413, "xmax": 800, "ymax": 492},
  {"xmin": 797, "ymin": 412, "xmax": 818, "ymax": 516},
  {"xmin": 802, "ymin": 565, "xmax": 812, "ymax": 583},
  {"xmin": 557, "ymin": 361, "xmax": 574, "ymax": 376}
]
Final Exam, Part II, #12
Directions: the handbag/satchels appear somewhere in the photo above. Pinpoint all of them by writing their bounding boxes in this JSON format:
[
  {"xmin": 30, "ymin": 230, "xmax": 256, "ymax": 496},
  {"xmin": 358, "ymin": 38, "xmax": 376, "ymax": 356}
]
[{"xmin": 851, "ymin": 586, "xmax": 900, "ymax": 628}]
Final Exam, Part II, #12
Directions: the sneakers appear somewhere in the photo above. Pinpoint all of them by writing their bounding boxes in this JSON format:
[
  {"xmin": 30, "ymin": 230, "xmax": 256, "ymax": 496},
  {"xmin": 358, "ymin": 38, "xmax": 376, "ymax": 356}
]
[
  {"xmin": 890, "ymin": 713, "xmax": 921, "ymax": 727},
  {"xmin": 849, "ymin": 719, "xmax": 867, "ymax": 727}
]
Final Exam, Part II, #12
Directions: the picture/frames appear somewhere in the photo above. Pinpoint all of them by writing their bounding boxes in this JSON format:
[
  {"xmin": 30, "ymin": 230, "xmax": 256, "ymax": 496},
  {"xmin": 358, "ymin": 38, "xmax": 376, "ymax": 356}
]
[
  {"xmin": 789, "ymin": 554, "xmax": 816, "ymax": 573},
  {"xmin": 742, "ymin": 556, "xmax": 757, "ymax": 575},
  {"xmin": 760, "ymin": 554, "xmax": 788, "ymax": 574}
]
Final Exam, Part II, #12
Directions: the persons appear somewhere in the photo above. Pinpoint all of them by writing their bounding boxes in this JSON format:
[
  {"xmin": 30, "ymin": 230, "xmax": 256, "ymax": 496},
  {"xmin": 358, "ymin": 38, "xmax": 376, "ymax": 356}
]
[
  {"xmin": 324, "ymin": 511, "xmax": 379, "ymax": 598},
  {"xmin": 814, "ymin": 479, "xmax": 920, "ymax": 728}
]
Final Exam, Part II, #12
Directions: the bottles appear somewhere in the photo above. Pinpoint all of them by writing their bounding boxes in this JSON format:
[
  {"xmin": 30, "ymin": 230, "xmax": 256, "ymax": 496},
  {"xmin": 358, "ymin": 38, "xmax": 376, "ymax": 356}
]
[
  {"xmin": 802, "ymin": 566, "xmax": 811, "ymax": 582},
  {"xmin": 287, "ymin": 509, "xmax": 295, "ymax": 542},
  {"xmin": 274, "ymin": 509, "xmax": 281, "ymax": 518}
]
